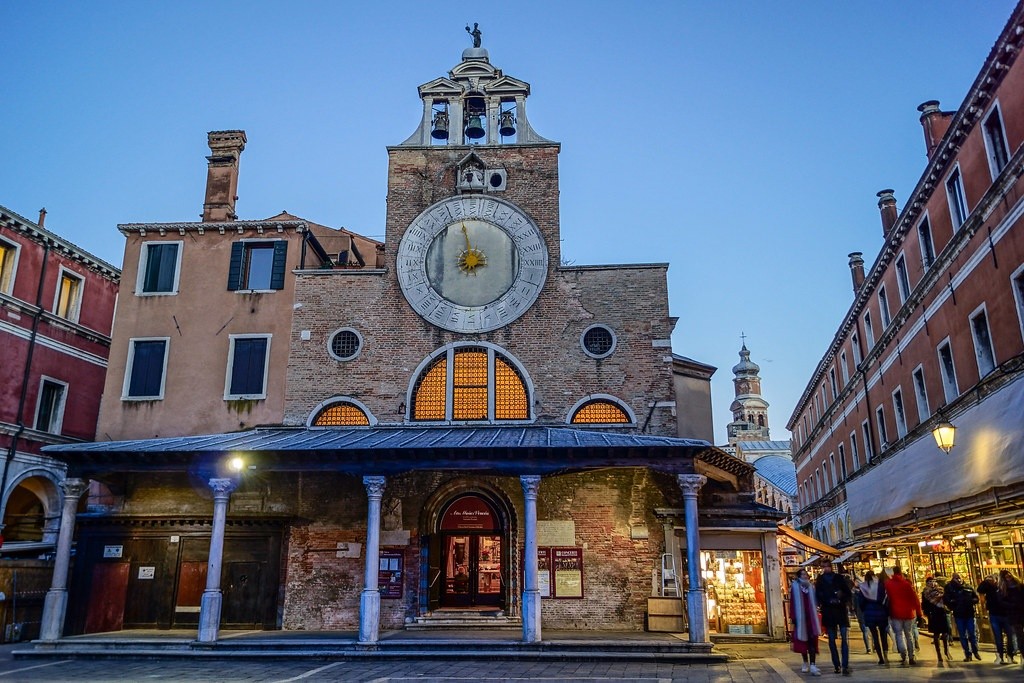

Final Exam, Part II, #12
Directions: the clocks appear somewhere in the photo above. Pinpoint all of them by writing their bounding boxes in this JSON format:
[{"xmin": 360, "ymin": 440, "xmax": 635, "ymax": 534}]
[{"xmin": 396, "ymin": 193, "xmax": 549, "ymax": 334}]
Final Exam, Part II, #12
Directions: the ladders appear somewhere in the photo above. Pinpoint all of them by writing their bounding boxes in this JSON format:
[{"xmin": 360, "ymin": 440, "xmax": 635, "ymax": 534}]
[{"xmin": 660, "ymin": 552, "xmax": 680, "ymax": 597}]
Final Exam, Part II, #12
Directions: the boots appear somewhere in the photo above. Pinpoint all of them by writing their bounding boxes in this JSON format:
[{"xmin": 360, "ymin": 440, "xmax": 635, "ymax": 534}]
[
  {"xmin": 936, "ymin": 649, "xmax": 943, "ymax": 661},
  {"xmin": 944, "ymin": 645, "xmax": 953, "ymax": 660}
]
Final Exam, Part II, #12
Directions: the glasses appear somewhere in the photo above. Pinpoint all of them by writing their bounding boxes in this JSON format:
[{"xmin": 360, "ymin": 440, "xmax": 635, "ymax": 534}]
[{"xmin": 821, "ymin": 565, "xmax": 828, "ymax": 568}]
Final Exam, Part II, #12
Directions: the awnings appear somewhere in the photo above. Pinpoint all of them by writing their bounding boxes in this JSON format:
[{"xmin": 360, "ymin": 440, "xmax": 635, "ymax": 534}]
[
  {"xmin": 800, "ymin": 555, "xmax": 820, "ymax": 565},
  {"xmin": 832, "ymin": 551, "xmax": 856, "ymax": 564}
]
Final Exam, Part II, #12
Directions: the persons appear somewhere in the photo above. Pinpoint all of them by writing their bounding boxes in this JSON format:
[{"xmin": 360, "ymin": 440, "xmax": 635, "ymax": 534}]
[
  {"xmin": 943, "ymin": 572, "xmax": 982, "ymax": 662},
  {"xmin": 858, "ymin": 570, "xmax": 889, "ymax": 666},
  {"xmin": 878, "ymin": 569, "xmax": 900, "ymax": 653},
  {"xmin": 850, "ymin": 578, "xmax": 874, "ymax": 653},
  {"xmin": 885, "ymin": 566, "xmax": 923, "ymax": 665},
  {"xmin": 976, "ymin": 573, "xmax": 1016, "ymax": 664},
  {"xmin": 454, "ymin": 567, "xmax": 469, "ymax": 592},
  {"xmin": 789, "ymin": 568, "xmax": 822, "ymax": 676},
  {"xmin": 816, "ymin": 560, "xmax": 855, "ymax": 676},
  {"xmin": 930, "ymin": 572, "xmax": 956, "ymax": 647},
  {"xmin": 1001, "ymin": 570, "xmax": 1024, "ymax": 664},
  {"xmin": 903, "ymin": 573, "xmax": 922, "ymax": 651},
  {"xmin": 921, "ymin": 577, "xmax": 953, "ymax": 668}
]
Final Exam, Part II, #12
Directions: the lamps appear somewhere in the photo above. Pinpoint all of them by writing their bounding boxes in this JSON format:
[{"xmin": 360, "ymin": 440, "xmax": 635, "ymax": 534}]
[{"xmin": 932, "ymin": 408, "xmax": 958, "ymax": 455}]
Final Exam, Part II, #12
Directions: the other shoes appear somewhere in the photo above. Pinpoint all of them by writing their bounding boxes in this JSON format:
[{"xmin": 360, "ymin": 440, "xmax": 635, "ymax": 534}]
[
  {"xmin": 878, "ymin": 657, "xmax": 884, "ymax": 664},
  {"xmin": 811, "ymin": 665, "xmax": 821, "ymax": 675},
  {"xmin": 834, "ymin": 666, "xmax": 841, "ymax": 673},
  {"xmin": 964, "ymin": 655, "xmax": 972, "ymax": 662},
  {"xmin": 909, "ymin": 660, "xmax": 917, "ymax": 665},
  {"xmin": 802, "ymin": 663, "xmax": 809, "ymax": 672},
  {"xmin": 867, "ymin": 649, "xmax": 871, "ymax": 654},
  {"xmin": 842, "ymin": 668, "xmax": 848, "ymax": 676},
  {"xmin": 974, "ymin": 653, "xmax": 981, "ymax": 660}
]
